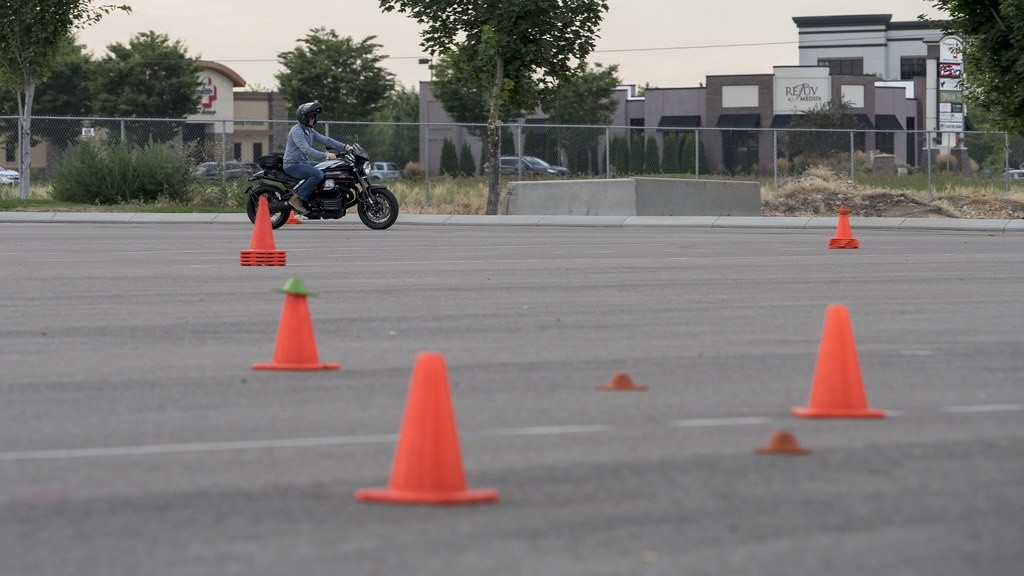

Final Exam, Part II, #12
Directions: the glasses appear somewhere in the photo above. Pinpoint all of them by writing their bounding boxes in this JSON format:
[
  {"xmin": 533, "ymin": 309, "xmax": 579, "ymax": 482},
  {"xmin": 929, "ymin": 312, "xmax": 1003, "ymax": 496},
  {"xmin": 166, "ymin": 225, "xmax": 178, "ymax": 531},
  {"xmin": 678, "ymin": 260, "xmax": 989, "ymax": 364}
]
[{"xmin": 308, "ymin": 112, "xmax": 318, "ymax": 120}]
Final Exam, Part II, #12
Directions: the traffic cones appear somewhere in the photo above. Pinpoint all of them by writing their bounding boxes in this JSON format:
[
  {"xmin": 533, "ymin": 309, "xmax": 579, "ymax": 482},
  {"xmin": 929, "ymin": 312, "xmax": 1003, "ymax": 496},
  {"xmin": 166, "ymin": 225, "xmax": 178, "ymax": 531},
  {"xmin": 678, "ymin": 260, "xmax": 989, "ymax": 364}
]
[
  {"xmin": 826, "ymin": 204, "xmax": 860, "ymax": 250},
  {"xmin": 249, "ymin": 276, "xmax": 341, "ymax": 371},
  {"xmin": 790, "ymin": 303, "xmax": 887, "ymax": 420},
  {"xmin": 352, "ymin": 350, "xmax": 501, "ymax": 505},
  {"xmin": 239, "ymin": 196, "xmax": 287, "ymax": 267},
  {"xmin": 285, "ymin": 208, "xmax": 302, "ymax": 224}
]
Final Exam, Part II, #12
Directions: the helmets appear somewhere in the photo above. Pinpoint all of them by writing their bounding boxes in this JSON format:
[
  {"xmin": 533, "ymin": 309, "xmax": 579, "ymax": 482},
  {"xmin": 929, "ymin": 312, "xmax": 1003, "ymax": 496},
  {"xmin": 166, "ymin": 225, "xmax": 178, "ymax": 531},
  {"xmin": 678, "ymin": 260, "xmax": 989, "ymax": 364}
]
[{"xmin": 296, "ymin": 103, "xmax": 322, "ymax": 126}]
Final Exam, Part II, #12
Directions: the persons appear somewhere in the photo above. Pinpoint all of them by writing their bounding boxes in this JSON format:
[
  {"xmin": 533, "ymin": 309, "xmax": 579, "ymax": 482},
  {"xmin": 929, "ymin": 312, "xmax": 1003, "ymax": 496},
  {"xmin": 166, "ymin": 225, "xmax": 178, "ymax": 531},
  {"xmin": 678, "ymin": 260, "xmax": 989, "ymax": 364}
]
[{"xmin": 283, "ymin": 101, "xmax": 353, "ymax": 214}]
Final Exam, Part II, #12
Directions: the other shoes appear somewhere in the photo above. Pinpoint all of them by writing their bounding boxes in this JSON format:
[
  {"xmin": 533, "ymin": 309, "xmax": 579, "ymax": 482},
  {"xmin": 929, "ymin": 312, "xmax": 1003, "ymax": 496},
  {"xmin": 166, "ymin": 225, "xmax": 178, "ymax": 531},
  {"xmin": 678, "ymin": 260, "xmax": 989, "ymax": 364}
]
[{"xmin": 289, "ymin": 194, "xmax": 306, "ymax": 213}]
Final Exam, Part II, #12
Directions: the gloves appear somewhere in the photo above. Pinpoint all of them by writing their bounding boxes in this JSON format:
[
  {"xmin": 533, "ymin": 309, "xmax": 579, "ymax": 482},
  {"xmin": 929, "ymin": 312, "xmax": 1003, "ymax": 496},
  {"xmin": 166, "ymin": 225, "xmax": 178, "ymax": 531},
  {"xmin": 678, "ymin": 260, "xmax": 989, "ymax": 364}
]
[
  {"xmin": 326, "ymin": 153, "xmax": 337, "ymax": 161},
  {"xmin": 345, "ymin": 144, "xmax": 354, "ymax": 153}
]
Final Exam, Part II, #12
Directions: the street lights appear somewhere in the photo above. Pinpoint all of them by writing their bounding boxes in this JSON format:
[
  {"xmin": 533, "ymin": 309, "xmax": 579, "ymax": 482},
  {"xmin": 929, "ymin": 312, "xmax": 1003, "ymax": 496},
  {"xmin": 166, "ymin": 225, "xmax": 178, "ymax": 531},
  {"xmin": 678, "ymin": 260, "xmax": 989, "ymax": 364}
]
[{"xmin": 417, "ymin": 56, "xmax": 434, "ymax": 83}]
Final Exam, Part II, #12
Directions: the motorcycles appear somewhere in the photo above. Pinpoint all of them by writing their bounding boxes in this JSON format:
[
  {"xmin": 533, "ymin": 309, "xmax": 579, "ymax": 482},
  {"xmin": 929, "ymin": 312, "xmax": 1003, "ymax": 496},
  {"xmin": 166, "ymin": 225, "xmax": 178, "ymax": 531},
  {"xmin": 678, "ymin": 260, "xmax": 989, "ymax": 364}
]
[{"xmin": 243, "ymin": 133, "xmax": 400, "ymax": 230}]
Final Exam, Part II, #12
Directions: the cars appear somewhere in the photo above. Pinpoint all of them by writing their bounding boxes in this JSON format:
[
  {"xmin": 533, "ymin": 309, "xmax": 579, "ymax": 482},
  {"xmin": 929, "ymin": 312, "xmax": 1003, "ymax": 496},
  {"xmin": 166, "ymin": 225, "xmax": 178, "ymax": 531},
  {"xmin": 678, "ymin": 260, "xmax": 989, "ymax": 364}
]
[
  {"xmin": 193, "ymin": 159, "xmax": 263, "ymax": 186},
  {"xmin": 0, "ymin": 165, "xmax": 20, "ymax": 186},
  {"xmin": 524, "ymin": 156, "xmax": 568, "ymax": 177},
  {"xmin": 482, "ymin": 155, "xmax": 557, "ymax": 178},
  {"xmin": 369, "ymin": 161, "xmax": 400, "ymax": 179}
]
